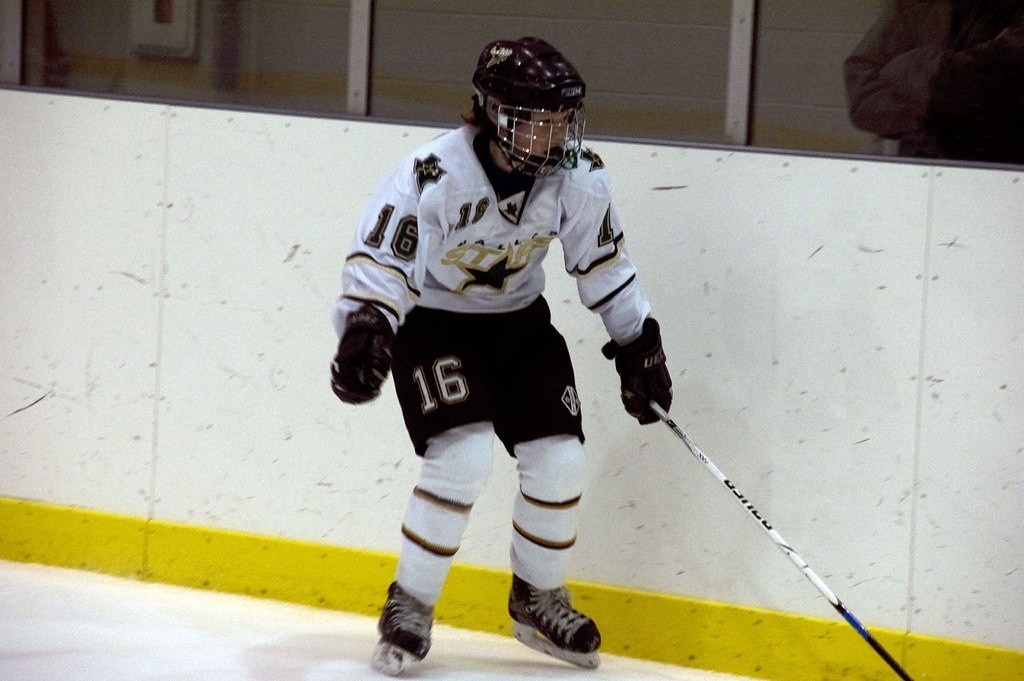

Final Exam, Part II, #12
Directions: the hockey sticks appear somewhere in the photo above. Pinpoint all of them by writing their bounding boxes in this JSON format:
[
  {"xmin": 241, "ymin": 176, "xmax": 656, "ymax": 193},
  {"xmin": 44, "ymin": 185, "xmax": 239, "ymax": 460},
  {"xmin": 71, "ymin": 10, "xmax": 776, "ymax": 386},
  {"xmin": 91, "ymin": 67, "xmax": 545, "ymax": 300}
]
[{"xmin": 651, "ymin": 399, "xmax": 918, "ymax": 681}]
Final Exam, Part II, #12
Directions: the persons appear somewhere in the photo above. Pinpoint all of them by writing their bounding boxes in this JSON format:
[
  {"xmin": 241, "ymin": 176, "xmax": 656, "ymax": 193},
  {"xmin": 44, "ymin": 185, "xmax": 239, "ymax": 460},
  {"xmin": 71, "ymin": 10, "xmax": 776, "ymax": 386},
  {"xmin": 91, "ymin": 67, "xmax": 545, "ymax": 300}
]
[
  {"xmin": 844, "ymin": 0, "xmax": 1024, "ymax": 167},
  {"xmin": 329, "ymin": 36, "xmax": 671, "ymax": 677}
]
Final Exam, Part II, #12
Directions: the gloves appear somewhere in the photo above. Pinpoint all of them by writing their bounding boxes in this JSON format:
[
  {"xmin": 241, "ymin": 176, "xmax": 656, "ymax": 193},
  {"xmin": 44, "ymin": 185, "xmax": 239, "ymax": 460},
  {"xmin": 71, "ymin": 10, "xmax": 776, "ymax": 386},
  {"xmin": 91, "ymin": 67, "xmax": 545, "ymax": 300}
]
[
  {"xmin": 601, "ymin": 317, "xmax": 673, "ymax": 426},
  {"xmin": 329, "ymin": 305, "xmax": 399, "ymax": 405}
]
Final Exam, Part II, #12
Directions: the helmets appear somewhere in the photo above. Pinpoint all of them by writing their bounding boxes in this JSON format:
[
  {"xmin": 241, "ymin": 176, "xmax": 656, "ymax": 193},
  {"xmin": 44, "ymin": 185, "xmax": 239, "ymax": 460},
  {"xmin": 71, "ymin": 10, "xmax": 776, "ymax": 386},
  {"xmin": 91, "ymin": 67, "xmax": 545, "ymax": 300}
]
[{"xmin": 471, "ymin": 36, "xmax": 587, "ymax": 180}]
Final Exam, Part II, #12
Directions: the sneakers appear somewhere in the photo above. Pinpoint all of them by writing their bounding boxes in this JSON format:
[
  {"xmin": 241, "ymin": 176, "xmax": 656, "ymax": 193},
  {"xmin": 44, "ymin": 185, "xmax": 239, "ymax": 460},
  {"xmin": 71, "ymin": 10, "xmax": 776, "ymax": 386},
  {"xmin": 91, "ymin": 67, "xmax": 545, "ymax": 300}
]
[
  {"xmin": 371, "ymin": 581, "xmax": 434, "ymax": 676},
  {"xmin": 507, "ymin": 572, "xmax": 602, "ymax": 669}
]
